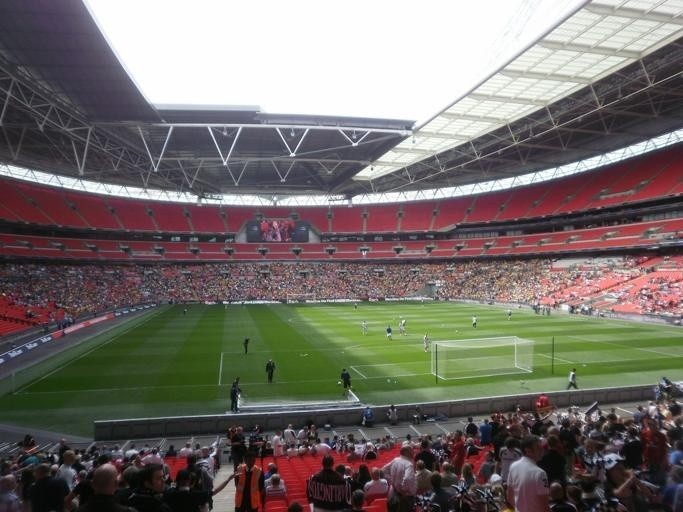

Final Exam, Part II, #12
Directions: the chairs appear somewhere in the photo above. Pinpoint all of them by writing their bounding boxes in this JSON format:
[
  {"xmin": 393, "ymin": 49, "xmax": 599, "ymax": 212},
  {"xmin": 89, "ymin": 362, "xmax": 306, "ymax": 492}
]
[
  {"xmin": 254, "ymin": 439, "xmax": 495, "ymax": 512},
  {"xmin": 532, "ymin": 256, "xmax": 682, "ymax": 322},
  {"xmin": 0, "ymin": 294, "xmax": 64, "ymax": 338},
  {"xmin": 165, "ymin": 456, "xmax": 187, "ymax": 480}
]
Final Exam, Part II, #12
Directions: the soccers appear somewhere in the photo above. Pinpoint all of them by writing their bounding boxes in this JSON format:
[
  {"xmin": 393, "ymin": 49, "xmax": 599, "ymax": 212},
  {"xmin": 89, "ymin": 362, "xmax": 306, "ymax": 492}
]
[
  {"xmin": 386, "ymin": 379, "xmax": 391, "ymax": 384},
  {"xmin": 337, "ymin": 381, "xmax": 341, "ymax": 385}
]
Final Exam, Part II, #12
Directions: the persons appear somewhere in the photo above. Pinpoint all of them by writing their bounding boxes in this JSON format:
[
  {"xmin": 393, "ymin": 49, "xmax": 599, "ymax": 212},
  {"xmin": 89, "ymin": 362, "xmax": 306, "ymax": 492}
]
[{"xmin": 1, "ymin": 255, "xmax": 682, "ymax": 511}]
